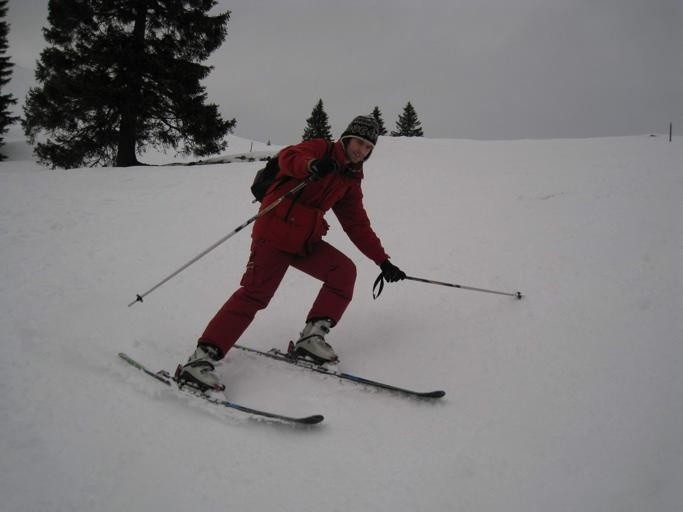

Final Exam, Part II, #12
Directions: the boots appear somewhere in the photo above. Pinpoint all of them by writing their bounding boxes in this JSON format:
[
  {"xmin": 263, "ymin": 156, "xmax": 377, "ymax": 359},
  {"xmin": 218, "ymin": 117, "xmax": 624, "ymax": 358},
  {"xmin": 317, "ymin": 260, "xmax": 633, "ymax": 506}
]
[
  {"xmin": 178, "ymin": 339, "xmax": 225, "ymax": 397},
  {"xmin": 294, "ymin": 317, "xmax": 339, "ymax": 364}
]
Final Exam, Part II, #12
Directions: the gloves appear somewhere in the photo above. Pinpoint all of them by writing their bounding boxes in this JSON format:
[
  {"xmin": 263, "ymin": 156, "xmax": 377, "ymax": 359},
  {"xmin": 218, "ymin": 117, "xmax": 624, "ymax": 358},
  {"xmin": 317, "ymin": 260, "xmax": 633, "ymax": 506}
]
[
  {"xmin": 306, "ymin": 155, "xmax": 341, "ymax": 178},
  {"xmin": 378, "ymin": 259, "xmax": 406, "ymax": 283}
]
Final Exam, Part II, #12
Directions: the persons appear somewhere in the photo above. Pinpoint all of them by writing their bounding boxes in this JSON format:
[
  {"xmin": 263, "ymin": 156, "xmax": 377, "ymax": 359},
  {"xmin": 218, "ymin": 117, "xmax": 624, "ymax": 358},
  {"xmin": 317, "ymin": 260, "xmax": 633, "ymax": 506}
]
[{"xmin": 177, "ymin": 114, "xmax": 408, "ymax": 394}]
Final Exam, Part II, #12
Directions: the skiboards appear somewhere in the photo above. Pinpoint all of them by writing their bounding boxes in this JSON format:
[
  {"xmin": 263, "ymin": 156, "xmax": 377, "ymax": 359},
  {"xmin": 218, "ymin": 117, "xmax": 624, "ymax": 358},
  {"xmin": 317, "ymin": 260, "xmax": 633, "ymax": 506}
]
[{"xmin": 118, "ymin": 343, "xmax": 446, "ymax": 425}]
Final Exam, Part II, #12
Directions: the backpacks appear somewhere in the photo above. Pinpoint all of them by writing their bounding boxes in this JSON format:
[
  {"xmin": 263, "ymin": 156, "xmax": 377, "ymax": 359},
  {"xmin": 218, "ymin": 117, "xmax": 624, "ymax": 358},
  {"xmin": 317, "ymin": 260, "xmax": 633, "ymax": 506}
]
[{"xmin": 251, "ymin": 134, "xmax": 335, "ymax": 205}]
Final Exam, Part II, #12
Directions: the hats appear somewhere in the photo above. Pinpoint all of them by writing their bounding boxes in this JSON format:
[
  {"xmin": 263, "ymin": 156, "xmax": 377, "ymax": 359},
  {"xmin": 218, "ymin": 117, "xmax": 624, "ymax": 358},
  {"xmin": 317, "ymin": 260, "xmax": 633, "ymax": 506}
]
[{"xmin": 339, "ymin": 115, "xmax": 380, "ymax": 148}]
